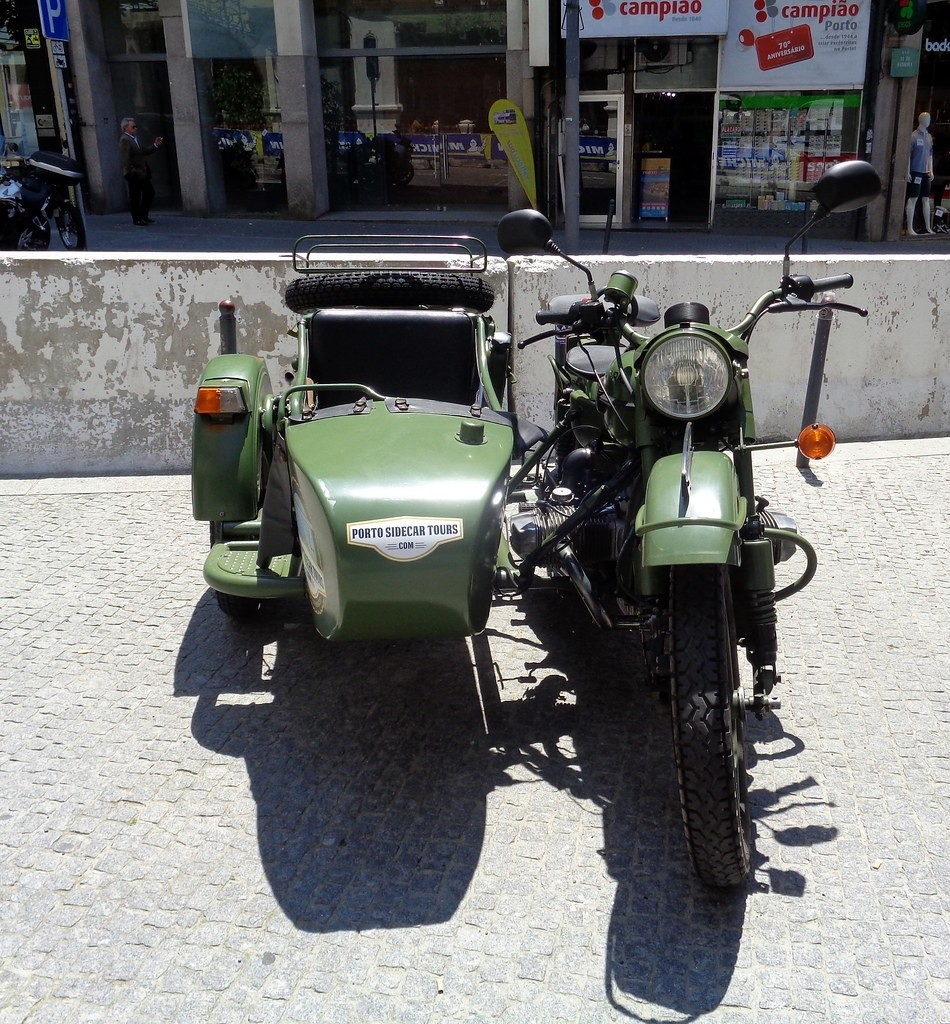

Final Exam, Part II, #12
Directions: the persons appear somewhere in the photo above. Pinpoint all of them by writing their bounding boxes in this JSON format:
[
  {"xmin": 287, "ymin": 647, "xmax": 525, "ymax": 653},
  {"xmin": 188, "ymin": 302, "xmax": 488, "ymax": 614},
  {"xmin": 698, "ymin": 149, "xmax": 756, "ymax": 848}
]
[
  {"xmin": 905, "ymin": 112, "xmax": 936, "ymax": 235},
  {"xmin": 0, "ymin": 134, "xmax": 18, "ymax": 157},
  {"xmin": 119, "ymin": 118, "xmax": 162, "ymax": 226},
  {"xmin": 408, "ymin": 115, "xmax": 425, "ymax": 133}
]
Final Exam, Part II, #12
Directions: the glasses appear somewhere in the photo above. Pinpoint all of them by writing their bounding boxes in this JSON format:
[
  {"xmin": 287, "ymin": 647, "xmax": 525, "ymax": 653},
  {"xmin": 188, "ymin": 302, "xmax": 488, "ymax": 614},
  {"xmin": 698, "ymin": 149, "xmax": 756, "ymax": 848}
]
[{"xmin": 131, "ymin": 125, "xmax": 137, "ymax": 129}]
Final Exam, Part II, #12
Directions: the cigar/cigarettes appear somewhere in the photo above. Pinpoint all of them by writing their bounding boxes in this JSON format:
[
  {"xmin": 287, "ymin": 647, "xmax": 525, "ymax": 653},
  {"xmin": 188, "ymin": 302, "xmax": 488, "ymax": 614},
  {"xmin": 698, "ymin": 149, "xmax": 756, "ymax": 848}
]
[{"xmin": 161, "ymin": 138, "xmax": 163, "ymax": 139}]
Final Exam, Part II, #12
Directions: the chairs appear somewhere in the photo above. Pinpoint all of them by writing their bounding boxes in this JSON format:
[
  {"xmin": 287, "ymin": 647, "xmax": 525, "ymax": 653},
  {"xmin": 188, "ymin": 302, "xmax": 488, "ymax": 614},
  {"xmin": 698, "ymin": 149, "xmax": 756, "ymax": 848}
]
[{"xmin": 308, "ymin": 308, "xmax": 474, "ymax": 408}]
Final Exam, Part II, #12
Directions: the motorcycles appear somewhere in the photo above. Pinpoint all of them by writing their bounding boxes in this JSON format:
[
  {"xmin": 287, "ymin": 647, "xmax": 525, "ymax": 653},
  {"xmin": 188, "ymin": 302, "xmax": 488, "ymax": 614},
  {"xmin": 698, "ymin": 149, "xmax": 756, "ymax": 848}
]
[
  {"xmin": 190, "ymin": 160, "xmax": 884, "ymax": 890},
  {"xmin": 0, "ymin": 139, "xmax": 86, "ymax": 251}
]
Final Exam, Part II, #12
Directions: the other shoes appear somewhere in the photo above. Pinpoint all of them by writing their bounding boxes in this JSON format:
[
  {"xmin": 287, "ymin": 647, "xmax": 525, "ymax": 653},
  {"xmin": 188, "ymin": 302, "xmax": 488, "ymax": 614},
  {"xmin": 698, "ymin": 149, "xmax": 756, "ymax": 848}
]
[{"xmin": 133, "ymin": 216, "xmax": 156, "ymax": 227}]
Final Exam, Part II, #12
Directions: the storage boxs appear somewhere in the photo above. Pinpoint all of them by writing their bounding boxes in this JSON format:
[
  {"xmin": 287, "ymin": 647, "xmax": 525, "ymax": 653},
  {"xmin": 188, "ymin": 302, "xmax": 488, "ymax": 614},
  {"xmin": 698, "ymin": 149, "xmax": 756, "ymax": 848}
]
[{"xmin": 29, "ymin": 151, "xmax": 84, "ymax": 186}]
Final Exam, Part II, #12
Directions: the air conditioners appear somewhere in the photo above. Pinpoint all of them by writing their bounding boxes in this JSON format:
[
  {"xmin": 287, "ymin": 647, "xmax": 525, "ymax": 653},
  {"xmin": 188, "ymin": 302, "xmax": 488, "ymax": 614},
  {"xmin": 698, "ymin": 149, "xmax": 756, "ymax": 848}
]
[{"xmin": 639, "ymin": 36, "xmax": 688, "ymax": 66}]
